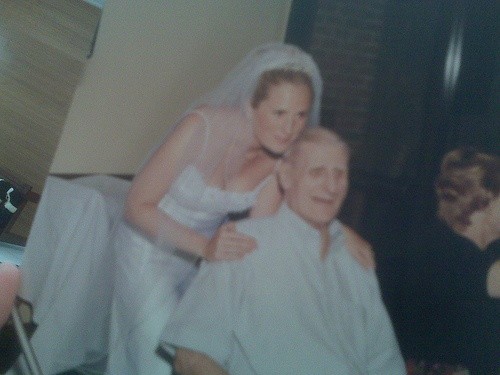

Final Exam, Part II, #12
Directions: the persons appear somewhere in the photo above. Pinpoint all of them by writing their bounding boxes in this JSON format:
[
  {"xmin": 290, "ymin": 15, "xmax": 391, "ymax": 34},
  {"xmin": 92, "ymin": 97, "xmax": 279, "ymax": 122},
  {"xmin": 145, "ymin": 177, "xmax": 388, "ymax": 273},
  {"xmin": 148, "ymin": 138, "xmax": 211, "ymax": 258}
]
[
  {"xmin": 108, "ymin": 38, "xmax": 324, "ymax": 375},
  {"xmin": 156, "ymin": 122, "xmax": 409, "ymax": 375},
  {"xmin": 379, "ymin": 146, "xmax": 499, "ymax": 375}
]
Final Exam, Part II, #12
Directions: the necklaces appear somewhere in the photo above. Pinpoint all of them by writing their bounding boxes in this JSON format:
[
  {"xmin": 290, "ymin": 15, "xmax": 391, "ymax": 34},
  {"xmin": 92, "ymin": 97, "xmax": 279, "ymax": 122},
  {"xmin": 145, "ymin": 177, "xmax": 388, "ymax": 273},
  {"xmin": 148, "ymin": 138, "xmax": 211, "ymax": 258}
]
[{"xmin": 224, "ymin": 120, "xmax": 277, "ymax": 195}]
